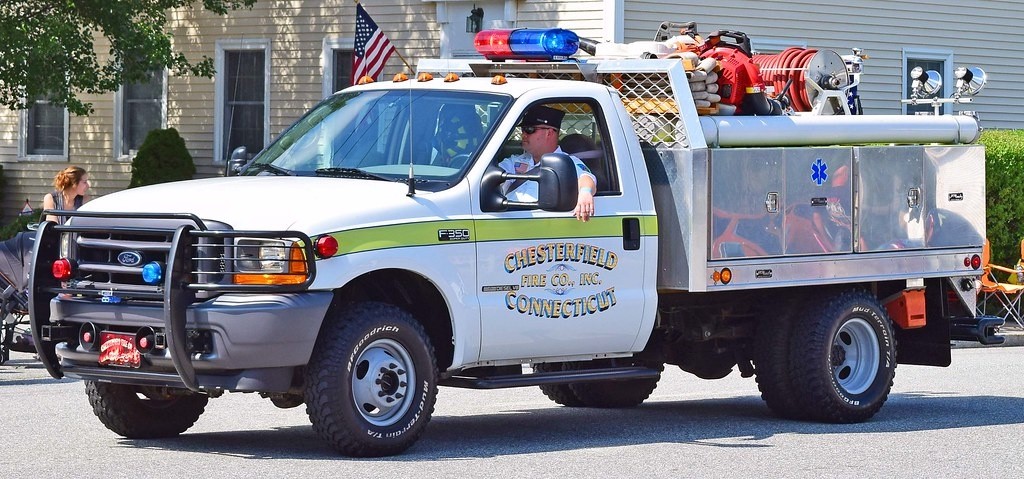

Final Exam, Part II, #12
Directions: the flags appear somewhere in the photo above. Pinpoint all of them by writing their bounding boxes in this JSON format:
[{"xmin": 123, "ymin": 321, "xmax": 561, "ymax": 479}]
[{"xmin": 350, "ymin": 3, "xmax": 396, "ymax": 85}]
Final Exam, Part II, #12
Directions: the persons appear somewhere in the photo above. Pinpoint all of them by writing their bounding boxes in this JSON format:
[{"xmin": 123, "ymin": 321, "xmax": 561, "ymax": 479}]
[
  {"xmin": 43, "ymin": 164, "xmax": 93, "ymax": 224},
  {"xmin": 497, "ymin": 105, "xmax": 599, "ymax": 223}
]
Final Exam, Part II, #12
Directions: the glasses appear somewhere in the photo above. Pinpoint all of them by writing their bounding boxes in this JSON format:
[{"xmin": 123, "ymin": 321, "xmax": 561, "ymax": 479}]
[{"xmin": 521, "ymin": 126, "xmax": 557, "ymax": 134}]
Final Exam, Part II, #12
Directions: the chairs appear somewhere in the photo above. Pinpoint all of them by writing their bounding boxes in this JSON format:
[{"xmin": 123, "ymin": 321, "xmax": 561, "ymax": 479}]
[
  {"xmin": 948, "ymin": 238, "xmax": 1024, "ymax": 330},
  {"xmin": 560, "ymin": 134, "xmax": 607, "ymax": 191}
]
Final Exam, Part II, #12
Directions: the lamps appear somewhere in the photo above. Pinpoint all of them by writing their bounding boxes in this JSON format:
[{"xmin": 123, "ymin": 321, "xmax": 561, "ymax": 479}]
[{"xmin": 465, "ymin": 3, "xmax": 484, "ymax": 34}]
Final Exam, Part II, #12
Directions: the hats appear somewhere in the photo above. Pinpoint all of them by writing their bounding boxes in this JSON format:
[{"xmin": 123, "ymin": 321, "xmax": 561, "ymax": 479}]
[{"xmin": 516, "ymin": 106, "xmax": 565, "ymax": 130}]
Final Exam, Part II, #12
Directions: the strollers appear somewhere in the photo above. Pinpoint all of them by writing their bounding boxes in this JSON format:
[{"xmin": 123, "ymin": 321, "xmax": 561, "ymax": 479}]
[{"xmin": 0, "ymin": 210, "xmax": 39, "ymax": 362}]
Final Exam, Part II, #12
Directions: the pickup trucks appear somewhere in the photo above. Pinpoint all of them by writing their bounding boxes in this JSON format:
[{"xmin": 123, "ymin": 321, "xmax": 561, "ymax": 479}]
[{"xmin": 30, "ymin": 23, "xmax": 1006, "ymax": 458}]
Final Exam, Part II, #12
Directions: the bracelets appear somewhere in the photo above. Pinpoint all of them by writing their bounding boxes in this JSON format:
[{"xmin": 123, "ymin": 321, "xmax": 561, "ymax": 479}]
[{"xmin": 581, "ymin": 187, "xmax": 592, "ymax": 193}]
